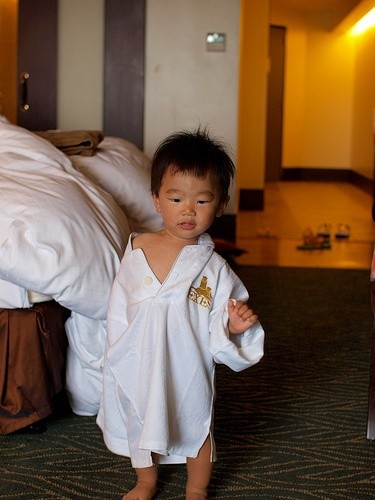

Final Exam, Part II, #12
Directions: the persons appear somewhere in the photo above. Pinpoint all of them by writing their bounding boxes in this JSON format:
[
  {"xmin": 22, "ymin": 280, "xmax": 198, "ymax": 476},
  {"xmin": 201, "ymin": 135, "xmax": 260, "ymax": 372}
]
[{"xmin": 103, "ymin": 124, "xmax": 265, "ymax": 500}]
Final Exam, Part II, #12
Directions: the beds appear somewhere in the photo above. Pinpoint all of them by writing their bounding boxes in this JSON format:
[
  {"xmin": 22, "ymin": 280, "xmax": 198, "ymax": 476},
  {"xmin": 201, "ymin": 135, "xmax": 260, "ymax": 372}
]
[{"xmin": 0, "ymin": 116, "xmax": 165, "ymax": 436}]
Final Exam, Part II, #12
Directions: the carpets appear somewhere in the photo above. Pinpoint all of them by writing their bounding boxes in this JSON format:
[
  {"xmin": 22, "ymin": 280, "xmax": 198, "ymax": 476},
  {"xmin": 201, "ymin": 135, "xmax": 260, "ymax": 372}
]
[{"xmin": 0, "ymin": 268, "xmax": 375, "ymax": 500}]
